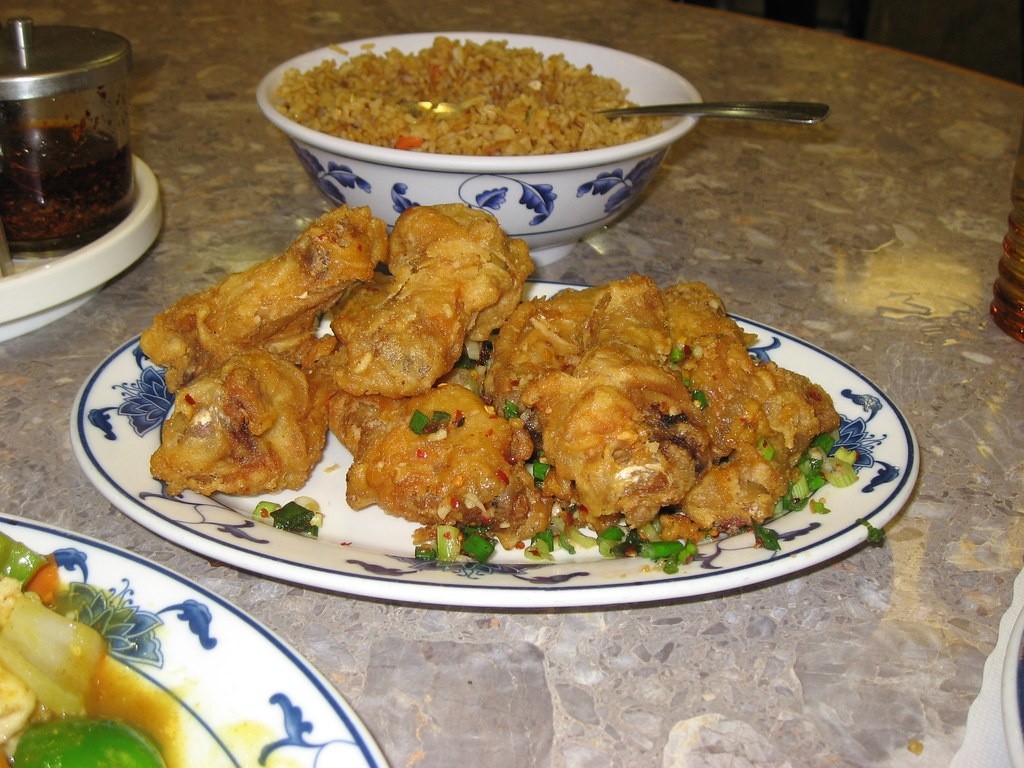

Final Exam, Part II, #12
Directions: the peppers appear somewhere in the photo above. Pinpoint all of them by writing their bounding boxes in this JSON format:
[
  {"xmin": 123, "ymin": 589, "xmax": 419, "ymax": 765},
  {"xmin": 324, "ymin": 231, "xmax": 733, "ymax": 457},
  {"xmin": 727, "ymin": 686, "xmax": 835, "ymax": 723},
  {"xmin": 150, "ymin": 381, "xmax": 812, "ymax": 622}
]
[{"xmin": 0, "ymin": 533, "xmax": 166, "ymax": 768}]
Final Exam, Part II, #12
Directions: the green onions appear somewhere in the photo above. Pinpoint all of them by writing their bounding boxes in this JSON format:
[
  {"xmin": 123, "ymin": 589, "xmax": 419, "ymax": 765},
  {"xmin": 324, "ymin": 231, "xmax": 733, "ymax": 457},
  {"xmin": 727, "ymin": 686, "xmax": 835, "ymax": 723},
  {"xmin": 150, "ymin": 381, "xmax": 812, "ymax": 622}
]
[{"xmin": 254, "ymin": 345, "xmax": 885, "ymax": 576}]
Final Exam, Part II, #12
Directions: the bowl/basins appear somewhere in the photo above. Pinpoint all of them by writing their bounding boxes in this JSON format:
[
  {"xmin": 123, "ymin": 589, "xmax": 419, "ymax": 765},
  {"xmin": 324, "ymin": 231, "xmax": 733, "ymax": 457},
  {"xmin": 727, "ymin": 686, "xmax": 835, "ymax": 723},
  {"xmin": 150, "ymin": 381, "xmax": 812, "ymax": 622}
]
[{"xmin": 255, "ymin": 32, "xmax": 702, "ymax": 263}]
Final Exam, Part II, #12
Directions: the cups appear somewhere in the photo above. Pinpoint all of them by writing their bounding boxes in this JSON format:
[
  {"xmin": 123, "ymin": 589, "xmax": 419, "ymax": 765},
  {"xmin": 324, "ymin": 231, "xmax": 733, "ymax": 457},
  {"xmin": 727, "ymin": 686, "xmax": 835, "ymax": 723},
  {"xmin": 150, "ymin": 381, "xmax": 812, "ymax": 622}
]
[{"xmin": 989, "ymin": 135, "xmax": 1024, "ymax": 342}]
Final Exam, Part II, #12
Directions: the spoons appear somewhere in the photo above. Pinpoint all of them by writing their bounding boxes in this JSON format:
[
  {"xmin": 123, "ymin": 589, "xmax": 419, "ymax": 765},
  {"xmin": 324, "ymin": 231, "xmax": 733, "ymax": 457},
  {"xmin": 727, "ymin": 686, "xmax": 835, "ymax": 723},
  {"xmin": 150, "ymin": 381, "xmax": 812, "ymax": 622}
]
[{"xmin": 415, "ymin": 100, "xmax": 829, "ymax": 125}]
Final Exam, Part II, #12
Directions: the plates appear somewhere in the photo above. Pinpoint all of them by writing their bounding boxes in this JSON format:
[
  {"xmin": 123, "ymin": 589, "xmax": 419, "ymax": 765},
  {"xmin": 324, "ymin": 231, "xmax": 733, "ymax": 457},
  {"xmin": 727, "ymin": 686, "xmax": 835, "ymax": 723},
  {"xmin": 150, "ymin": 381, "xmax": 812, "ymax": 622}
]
[
  {"xmin": 1000, "ymin": 606, "xmax": 1024, "ymax": 768},
  {"xmin": 71, "ymin": 284, "xmax": 920, "ymax": 611},
  {"xmin": 0, "ymin": 512, "xmax": 389, "ymax": 768}
]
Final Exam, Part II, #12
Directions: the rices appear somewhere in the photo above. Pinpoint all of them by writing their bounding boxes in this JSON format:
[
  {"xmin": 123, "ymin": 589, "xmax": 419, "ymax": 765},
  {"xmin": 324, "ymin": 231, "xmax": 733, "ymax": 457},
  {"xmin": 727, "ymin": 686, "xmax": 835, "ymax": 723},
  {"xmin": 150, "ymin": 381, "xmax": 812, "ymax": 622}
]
[{"xmin": 273, "ymin": 34, "xmax": 669, "ymax": 155}]
